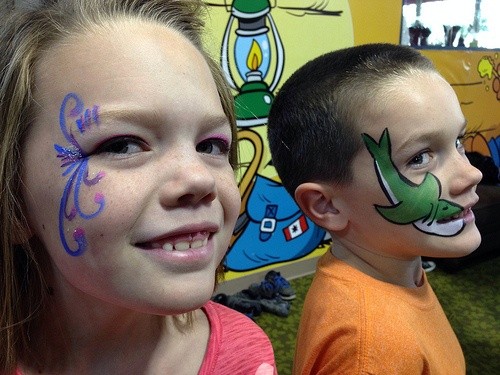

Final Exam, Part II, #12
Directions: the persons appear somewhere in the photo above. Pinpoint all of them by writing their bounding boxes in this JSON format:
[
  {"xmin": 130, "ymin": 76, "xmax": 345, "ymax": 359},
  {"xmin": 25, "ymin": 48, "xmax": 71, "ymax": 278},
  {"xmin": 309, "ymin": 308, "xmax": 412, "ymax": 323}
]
[
  {"xmin": 0, "ymin": 0, "xmax": 279, "ymax": 375},
  {"xmin": 267, "ymin": 42, "xmax": 483, "ymax": 375}
]
